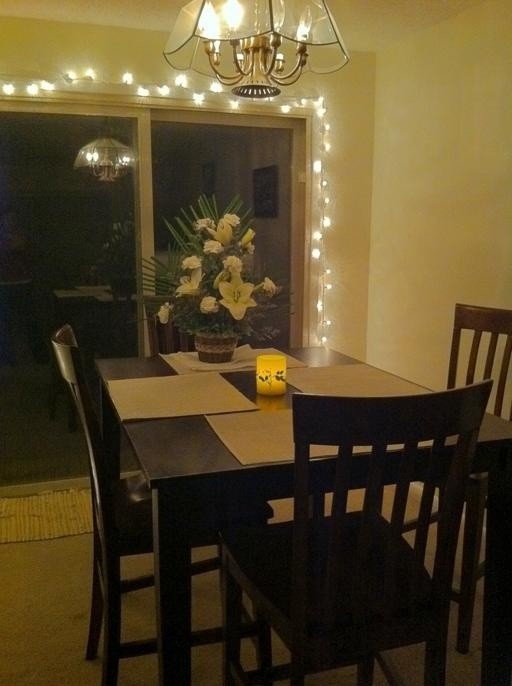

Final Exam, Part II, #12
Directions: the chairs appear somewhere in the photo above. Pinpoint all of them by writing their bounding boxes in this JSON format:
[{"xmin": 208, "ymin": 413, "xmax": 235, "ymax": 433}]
[
  {"xmin": 221, "ymin": 376, "xmax": 493, "ymax": 685},
  {"xmin": 148, "ymin": 306, "xmax": 203, "ymax": 349},
  {"xmin": 51, "ymin": 327, "xmax": 272, "ymax": 682},
  {"xmin": 368, "ymin": 299, "xmax": 510, "ymax": 655}
]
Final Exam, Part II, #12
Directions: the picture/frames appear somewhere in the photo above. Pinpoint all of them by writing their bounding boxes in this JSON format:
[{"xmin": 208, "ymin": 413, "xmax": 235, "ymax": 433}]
[{"xmin": 250, "ymin": 165, "xmax": 279, "ymax": 219}]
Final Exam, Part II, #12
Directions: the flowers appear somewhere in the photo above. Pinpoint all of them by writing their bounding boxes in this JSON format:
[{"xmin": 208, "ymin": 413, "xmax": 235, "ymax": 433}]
[{"xmin": 124, "ymin": 187, "xmax": 297, "ymax": 342}]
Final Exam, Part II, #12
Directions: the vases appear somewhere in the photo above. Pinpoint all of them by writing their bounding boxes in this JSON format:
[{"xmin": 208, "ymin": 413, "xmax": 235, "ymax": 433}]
[{"xmin": 188, "ymin": 329, "xmax": 238, "ymax": 362}]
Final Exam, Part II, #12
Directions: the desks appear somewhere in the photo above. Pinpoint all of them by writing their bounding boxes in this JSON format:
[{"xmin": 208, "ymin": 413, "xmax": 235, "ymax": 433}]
[{"xmin": 88, "ymin": 347, "xmax": 510, "ymax": 685}]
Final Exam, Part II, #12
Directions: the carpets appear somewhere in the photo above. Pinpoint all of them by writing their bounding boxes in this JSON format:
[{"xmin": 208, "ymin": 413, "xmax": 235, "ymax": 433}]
[{"xmin": 0, "ymin": 487, "xmax": 93, "ymax": 542}]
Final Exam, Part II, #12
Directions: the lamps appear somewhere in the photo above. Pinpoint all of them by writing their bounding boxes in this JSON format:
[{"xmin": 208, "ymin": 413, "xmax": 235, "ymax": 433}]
[
  {"xmin": 75, "ymin": 113, "xmax": 135, "ymax": 185},
  {"xmin": 157, "ymin": 0, "xmax": 351, "ymax": 101}
]
